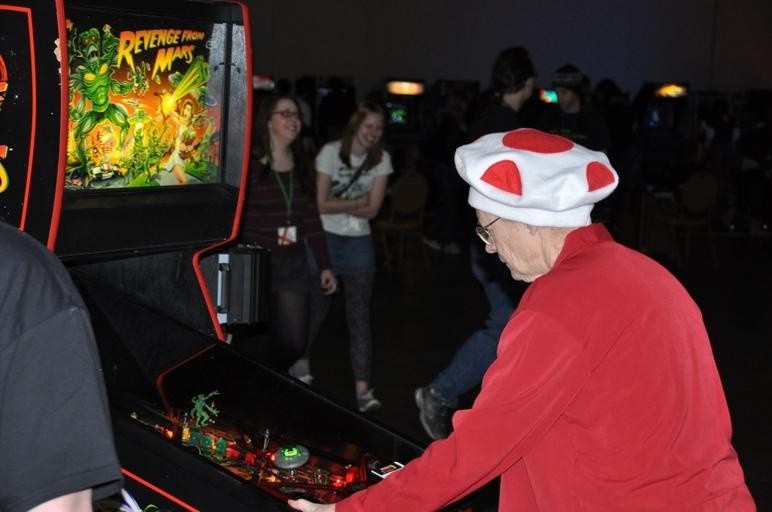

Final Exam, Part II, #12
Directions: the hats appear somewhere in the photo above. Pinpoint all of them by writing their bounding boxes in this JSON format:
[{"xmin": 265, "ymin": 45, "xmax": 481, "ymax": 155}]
[{"xmin": 454, "ymin": 127, "xmax": 621, "ymax": 228}]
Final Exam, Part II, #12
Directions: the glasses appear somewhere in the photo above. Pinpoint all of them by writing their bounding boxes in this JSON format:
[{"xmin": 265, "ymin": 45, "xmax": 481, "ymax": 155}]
[
  {"xmin": 271, "ymin": 111, "xmax": 304, "ymax": 118},
  {"xmin": 475, "ymin": 217, "xmax": 501, "ymax": 246}
]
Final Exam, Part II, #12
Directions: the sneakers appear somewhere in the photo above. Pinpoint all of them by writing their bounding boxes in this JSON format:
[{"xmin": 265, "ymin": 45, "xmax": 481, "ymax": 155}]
[
  {"xmin": 415, "ymin": 383, "xmax": 449, "ymax": 440},
  {"xmin": 357, "ymin": 391, "xmax": 382, "ymax": 413}
]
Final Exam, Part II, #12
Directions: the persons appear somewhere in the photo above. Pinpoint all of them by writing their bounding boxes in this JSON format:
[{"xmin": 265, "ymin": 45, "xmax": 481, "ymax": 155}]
[
  {"xmin": 290, "ymin": 102, "xmax": 397, "ymax": 416},
  {"xmin": 0, "ymin": 220, "xmax": 128, "ymax": 510},
  {"xmin": 544, "ymin": 62, "xmax": 617, "ymax": 151},
  {"xmin": 414, "ymin": 45, "xmax": 557, "ymax": 440},
  {"xmin": 252, "ymin": 93, "xmax": 339, "ymax": 374},
  {"xmin": 285, "ymin": 126, "xmax": 757, "ymax": 511}
]
